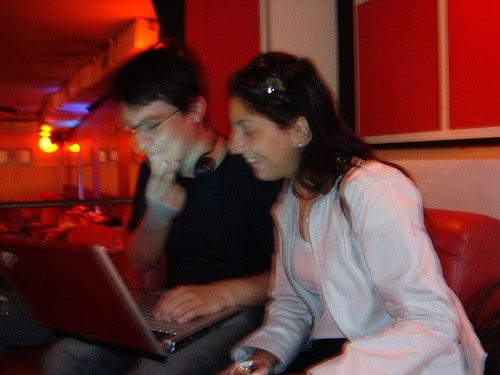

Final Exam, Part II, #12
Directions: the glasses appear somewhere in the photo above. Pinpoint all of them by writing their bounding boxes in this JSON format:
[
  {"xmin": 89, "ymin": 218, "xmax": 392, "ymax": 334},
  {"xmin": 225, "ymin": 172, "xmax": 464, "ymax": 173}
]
[
  {"xmin": 126, "ymin": 108, "xmax": 180, "ymax": 136},
  {"xmin": 251, "ymin": 54, "xmax": 287, "ymax": 101}
]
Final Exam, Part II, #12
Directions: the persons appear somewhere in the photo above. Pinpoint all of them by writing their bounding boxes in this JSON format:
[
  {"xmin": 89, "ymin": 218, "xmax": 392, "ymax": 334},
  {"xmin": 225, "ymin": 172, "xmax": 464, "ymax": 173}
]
[
  {"xmin": 40, "ymin": 46, "xmax": 277, "ymax": 375},
  {"xmin": 216, "ymin": 52, "xmax": 488, "ymax": 375}
]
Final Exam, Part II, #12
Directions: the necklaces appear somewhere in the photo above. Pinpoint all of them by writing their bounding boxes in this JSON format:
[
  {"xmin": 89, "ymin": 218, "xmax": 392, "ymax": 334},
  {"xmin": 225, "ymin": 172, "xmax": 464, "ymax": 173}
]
[{"xmin": 291, "ymin": 176, "xmax": 326, "ymax": 223}]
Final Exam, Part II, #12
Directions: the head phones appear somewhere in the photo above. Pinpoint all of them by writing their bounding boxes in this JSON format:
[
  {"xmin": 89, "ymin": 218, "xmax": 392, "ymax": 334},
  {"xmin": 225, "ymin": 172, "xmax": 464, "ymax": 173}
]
[{"xmin": 190, "ymin": 128, "xmax": 219, "ymax": 181}]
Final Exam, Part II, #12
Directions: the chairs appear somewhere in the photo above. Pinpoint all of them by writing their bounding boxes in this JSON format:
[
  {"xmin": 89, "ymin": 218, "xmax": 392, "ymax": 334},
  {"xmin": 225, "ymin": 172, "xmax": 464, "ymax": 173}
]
[{"xmin": 422, "ymin": 204, "xmax": 500, "ymax": 375}]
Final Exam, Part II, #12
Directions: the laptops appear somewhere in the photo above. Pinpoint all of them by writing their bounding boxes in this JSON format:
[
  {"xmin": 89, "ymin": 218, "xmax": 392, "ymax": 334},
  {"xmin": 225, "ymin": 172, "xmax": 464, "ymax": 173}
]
[{"xmin": 1, "ymin": 237, "xmax": 245, "ymax": 363}]
